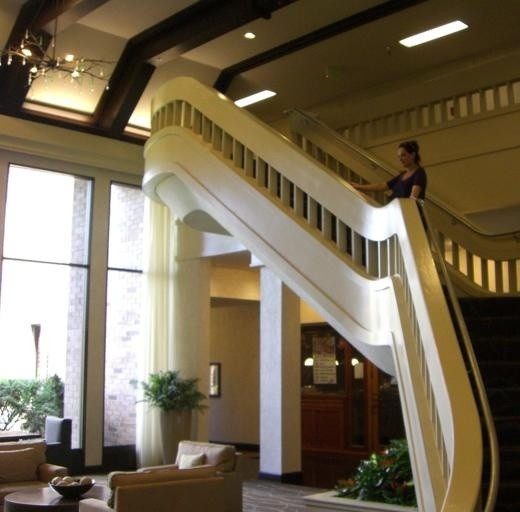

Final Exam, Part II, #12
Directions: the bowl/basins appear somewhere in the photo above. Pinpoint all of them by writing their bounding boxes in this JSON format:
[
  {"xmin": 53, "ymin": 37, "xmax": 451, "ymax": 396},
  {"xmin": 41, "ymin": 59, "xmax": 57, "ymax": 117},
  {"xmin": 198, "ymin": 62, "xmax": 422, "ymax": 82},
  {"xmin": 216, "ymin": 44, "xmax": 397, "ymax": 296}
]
[{"xmin": 49, "ymin": 482, "xmax": 97, "ymax": 496}]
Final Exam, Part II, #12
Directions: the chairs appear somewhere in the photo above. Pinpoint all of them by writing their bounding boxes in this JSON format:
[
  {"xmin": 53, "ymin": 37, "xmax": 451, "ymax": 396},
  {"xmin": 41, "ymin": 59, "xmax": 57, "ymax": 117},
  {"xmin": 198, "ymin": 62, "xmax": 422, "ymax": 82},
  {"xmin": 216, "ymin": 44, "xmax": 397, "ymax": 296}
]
[{"xmin": 0, "ymin": 413, "xmax": 244, "ymax": 511}]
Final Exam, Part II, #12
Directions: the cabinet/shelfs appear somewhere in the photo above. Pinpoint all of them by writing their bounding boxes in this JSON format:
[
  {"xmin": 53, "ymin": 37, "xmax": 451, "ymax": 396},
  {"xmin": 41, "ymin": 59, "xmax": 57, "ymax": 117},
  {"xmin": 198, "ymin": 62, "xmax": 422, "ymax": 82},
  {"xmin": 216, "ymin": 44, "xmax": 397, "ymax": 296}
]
[{"xmin": 297, "ymin": 317, "xmax": 407, "ymax": 489}]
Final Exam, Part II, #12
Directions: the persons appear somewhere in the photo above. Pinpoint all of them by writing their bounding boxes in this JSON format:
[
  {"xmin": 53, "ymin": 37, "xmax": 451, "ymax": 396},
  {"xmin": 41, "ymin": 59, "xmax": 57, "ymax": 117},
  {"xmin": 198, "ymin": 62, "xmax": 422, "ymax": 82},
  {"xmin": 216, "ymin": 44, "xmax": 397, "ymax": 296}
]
[{"xmin": 350, "ymin": 139, "xmax": 427, "ymax": 232}]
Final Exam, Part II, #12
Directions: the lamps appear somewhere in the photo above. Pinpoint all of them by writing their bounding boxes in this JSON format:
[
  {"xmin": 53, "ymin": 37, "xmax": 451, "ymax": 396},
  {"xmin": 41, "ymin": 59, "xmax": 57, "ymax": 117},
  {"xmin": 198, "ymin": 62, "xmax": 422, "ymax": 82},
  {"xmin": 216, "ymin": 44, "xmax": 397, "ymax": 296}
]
[{"xmin": 0, "ymin": 2, "xmax": 120, "ymax": 100}]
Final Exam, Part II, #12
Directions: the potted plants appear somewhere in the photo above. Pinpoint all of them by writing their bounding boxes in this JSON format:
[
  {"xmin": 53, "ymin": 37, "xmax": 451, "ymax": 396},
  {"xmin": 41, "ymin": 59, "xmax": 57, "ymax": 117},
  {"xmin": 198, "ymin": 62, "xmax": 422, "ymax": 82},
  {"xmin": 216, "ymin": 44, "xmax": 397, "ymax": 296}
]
[
  {"xmin": 299, "ymin": 435, "xmax": 422, "ymax": 511},
  {"xmin": 131, "ymin": 368, "xmax": 211, "ymax": 466}
]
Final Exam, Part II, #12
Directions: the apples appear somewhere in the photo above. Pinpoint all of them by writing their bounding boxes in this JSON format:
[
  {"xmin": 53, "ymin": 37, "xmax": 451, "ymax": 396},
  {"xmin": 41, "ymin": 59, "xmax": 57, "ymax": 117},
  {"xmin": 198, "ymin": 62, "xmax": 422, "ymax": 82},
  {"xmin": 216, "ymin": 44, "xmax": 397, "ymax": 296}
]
[{"xmin": 53, "ymin": 475, "xmax": 92, "ymax": 486}]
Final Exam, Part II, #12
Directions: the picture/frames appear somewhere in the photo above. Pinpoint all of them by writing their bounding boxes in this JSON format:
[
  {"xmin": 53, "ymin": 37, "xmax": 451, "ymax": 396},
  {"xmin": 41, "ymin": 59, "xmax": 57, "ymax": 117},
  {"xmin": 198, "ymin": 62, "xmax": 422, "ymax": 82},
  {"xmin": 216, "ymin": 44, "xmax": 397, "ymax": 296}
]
[{"xmin": 207, "ymin": 360, "xmax": 222, "ymax": 397}]
